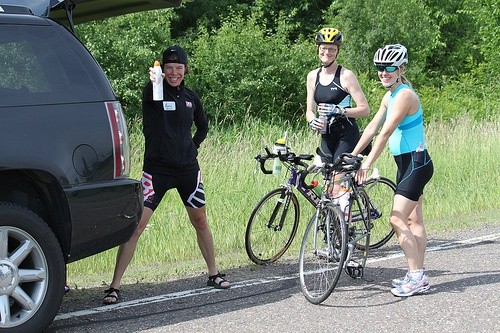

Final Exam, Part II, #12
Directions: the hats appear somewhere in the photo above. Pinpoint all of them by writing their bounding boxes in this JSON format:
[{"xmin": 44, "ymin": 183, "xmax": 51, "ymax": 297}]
[{"xmin": 162, "ymin": 46, "xmax": 187, "ymax": 65}]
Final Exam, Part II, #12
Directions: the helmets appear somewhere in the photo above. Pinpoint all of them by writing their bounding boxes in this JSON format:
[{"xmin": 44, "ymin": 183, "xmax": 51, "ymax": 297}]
[
  {"xmin": 373, "ymin": 44, "xmax": 408, "ymax": 68},
  {"xmin": 315, "ymin": 27, "xmax": 342, "ymax": 46}
]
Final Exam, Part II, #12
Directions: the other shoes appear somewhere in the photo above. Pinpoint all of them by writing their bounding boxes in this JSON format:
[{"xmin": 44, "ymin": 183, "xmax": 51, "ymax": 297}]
[{"xmin": 319, "ymin": 247, "xmax": 341, "ymax": 261}]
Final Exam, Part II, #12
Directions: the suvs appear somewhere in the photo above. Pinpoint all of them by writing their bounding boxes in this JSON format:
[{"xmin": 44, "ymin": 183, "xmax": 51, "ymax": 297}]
[{"xmin": 0, "ymin": 0, "xmax": 182, "ymax": 333}]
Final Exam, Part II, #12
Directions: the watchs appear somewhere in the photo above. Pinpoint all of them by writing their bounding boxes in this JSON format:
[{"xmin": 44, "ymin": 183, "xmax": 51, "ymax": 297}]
[{"xmin": 342, "ymin": 107, "xmax": 346, "ymax": 116}]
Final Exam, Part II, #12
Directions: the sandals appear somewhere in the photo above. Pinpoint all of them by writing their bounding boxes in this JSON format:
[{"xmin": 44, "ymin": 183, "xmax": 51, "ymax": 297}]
[
  {"xmin": 103, "ymin": 287, "xmax": 120, "ymax": 305},
  {"xmin": 207, "ymin": 272, "xmax": 231, "ymax": 289}
]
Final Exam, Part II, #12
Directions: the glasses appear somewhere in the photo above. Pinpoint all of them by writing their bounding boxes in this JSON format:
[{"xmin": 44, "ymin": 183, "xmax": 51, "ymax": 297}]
[{"xmin": 376, "ymin": 65, "xmax": 402, "ymax": 72}]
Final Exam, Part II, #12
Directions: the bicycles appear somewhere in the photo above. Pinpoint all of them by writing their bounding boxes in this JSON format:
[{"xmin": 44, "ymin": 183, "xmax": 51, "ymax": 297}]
[{"xmin": 245, "ymin": 130, "xmax": 399, "ymax": 305}]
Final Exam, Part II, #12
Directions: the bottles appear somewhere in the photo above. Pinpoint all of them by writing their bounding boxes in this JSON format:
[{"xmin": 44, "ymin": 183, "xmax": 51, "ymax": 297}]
[
  {"xmin": 273, "ymin": 139, "xmax": 286, "ymax": 174},
  {"xmin": 339, "ymin": 186, "xmax": 351, "ymax": 220},
  {"xmin": 317, "ymin": 104, "xmax": 328, "ymax": 133},
  {"xmin": 153, "ymin": 61, "xmax": 163, "ymax": 100},
  {"xmin": 311, "ymin": 179, "xmax": 323, "ymax": 197}
]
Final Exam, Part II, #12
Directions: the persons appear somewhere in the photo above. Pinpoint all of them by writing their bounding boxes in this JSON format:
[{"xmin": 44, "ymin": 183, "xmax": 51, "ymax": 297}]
[
  {"xmin": 102, "ymin": 46, "xmax": 231, "ymax": 304},
  {"xmin": 306, "ymin": 28, "xmax": 371, "ymax": 258},
  {"xmin": 343, "ymin": 43, "xmax": 434, "ymax": 296}
]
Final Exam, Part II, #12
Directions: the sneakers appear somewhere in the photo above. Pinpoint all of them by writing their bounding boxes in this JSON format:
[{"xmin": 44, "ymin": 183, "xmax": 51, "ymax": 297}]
[
  {"xmin": 391, "ymin": 274, "xmax": 430, "ymax": 297},
  {"xmin": 392, "ymin": 274, "xmax": 410, "ymax": 287}
]
